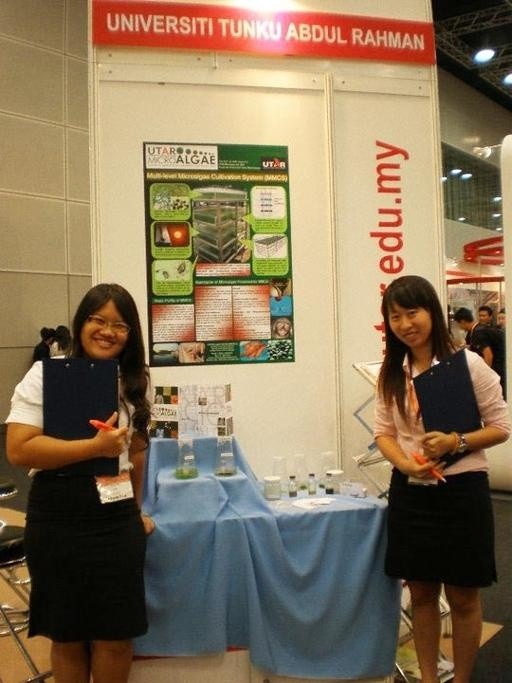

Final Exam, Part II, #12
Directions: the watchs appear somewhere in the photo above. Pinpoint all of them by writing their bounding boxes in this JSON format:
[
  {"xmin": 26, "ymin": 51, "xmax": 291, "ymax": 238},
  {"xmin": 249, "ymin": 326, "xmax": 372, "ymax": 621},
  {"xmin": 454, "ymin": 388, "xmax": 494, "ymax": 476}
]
[{"xmin": 457, "ymin": 431, "xmax": 469, "ymax": 454}]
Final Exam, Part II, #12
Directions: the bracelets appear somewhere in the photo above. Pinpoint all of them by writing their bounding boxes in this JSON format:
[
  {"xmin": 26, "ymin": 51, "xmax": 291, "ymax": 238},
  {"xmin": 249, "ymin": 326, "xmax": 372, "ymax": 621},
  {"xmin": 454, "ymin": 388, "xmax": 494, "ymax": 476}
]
[{"xmin": 448, "ymin": 431, "xmax": 458, "ymax": 457}]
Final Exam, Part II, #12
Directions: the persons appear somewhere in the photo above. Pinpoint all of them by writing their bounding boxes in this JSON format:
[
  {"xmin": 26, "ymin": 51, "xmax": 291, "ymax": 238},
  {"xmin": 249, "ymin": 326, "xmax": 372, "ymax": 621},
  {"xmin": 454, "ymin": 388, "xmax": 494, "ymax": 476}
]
[
  {"xmin": 48, "ymin": 324, "xmax": 74, "ymax": 356},
  {"xmin": 32, "ymin": 325, "xmax": 55, "ymax": 362},
  {"xmin": 451, "ymin": 304, "xmax": 506, "ymax": 400},
  {"xmin": 478, "ymin": 306, "xmax": 501, "ymax": 329},
  {"xmin": 495, "ymin": 307, "xmax": 506, "ymax": 328},
  {"xmin": 4, "ymin": 282, "xmax": 155, "ymax": 683},
  {"xmin": 370, "ymin": 275, "xmax": 512, "ymax": 682}
]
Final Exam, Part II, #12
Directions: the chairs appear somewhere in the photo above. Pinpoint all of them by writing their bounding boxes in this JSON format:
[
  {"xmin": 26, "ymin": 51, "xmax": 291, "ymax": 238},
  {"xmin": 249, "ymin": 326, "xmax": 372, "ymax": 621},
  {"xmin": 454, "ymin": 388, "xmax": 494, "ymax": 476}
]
[{"xmin": 0, "ymin": 481, "xmax": 54, "ymax": 683}]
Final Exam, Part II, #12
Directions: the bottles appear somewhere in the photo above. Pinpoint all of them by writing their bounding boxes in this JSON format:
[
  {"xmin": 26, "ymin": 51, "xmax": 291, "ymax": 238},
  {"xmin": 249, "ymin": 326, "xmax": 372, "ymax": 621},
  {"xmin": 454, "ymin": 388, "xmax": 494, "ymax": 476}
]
[
  {"xmin": 308, "ymin": 474, "xmax": 316, "ymax": 495},
  {"xmin": 175, "ymin": 438, "xmax": 198, "ymax": 479},
  {"xmin": 289, "ymin": 476, "xmax": 297, "ymax": 498},
  {"xmin": 215, "ymin": 436, "xmax": 237, "ymax": 475},
  {"xmin": 326, "ymin": 472, "xmax": 333, "ymax": 493}
]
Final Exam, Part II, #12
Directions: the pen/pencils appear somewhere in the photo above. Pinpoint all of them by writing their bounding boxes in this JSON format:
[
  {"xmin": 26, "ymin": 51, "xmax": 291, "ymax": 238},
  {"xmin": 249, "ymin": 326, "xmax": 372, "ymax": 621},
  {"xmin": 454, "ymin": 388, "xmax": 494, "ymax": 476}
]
[
  {"xmin": 89, "ymin": 419, "xmax": 133, "ymax": 439},
  {"xmin": 410, "ymin": 450, "xmax": 447, "ymax": 484}
]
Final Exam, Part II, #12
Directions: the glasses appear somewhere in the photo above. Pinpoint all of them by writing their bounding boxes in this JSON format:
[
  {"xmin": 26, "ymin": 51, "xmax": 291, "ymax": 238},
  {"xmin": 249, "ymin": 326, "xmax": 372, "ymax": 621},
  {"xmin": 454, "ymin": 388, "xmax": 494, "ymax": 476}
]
[{"xmin": 88, "ymin": 314, "xmax": 131, "ymax": 335}]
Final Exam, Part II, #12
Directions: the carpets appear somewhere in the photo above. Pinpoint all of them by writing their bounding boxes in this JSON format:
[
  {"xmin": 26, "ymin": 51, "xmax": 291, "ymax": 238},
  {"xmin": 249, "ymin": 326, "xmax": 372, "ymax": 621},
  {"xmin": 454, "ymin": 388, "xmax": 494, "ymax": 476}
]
[{"xmin": 0, "ymin": 506, "xmax": 504, "ymax": 683}]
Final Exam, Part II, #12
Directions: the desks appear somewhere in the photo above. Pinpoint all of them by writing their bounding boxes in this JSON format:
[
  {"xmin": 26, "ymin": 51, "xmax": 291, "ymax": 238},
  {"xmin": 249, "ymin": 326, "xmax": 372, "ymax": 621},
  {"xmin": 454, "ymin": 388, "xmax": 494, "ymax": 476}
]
[{"xmin": 122, "ymin": 436, "xmax": 403, "ymax": 683}]
